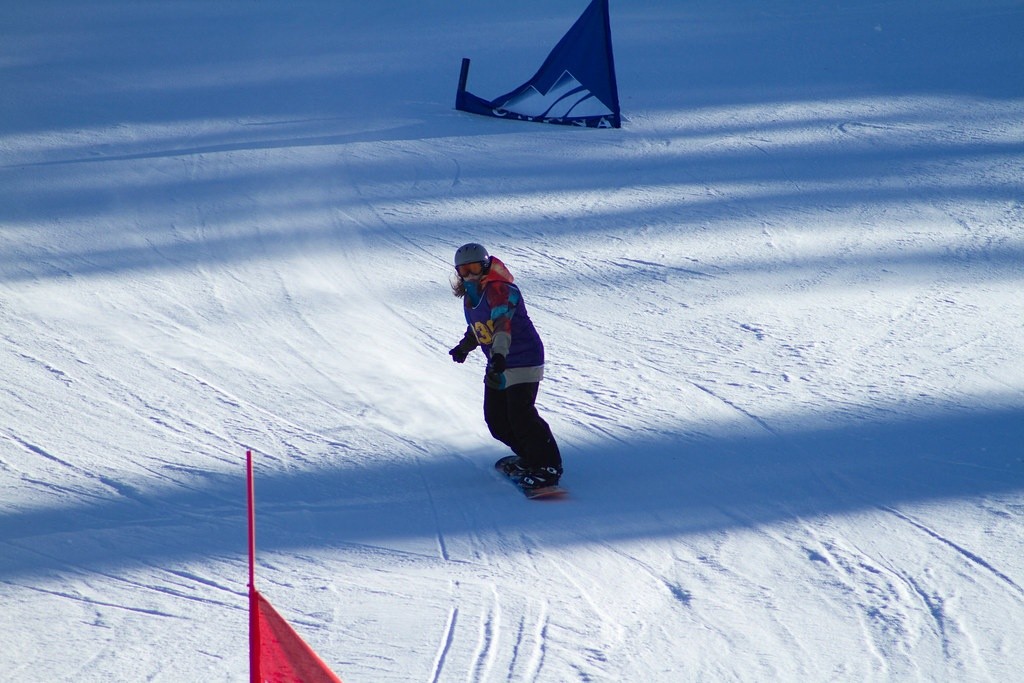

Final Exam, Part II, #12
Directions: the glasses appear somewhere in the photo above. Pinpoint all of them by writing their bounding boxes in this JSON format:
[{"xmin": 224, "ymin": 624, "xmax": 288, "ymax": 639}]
[{"xmin": 458, "ymin": 262, "xmax": 484, "ymax": 278}]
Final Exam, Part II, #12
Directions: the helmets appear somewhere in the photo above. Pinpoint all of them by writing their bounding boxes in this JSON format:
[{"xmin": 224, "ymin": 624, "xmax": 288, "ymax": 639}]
[{"xmin": 455, "ymin": 243, "xmax": 490, "ymax": 274}]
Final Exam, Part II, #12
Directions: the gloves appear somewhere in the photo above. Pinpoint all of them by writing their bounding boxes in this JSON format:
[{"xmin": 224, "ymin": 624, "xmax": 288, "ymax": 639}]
[
  {"xmin": 490, "ymin": 354, "xmax": 507, "ymax": 374},
  {"xmin": 449, "ymin": 343, "xmax": 471, "ymax": 363}
]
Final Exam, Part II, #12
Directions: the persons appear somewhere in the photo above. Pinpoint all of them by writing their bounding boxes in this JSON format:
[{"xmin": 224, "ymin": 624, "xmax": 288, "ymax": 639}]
[{"xmin": 448, "ymin": 243, "xmax": 562, "ymax": 489}]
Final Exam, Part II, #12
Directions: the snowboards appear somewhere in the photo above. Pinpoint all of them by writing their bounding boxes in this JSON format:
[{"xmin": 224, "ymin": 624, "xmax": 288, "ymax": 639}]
[{"xmin": 494, "ymin": 455, "xmax": 570, "ymax": 500}]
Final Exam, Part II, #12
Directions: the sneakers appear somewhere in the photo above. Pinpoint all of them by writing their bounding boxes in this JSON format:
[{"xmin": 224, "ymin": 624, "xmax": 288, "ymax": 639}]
[
  {"xmin": 524, "ymin": 465, "xmax": 564, "ymax": 486},
  {"xmin": 503, "ymin": 459, "xmax": 532, "ymax": 475}
]
